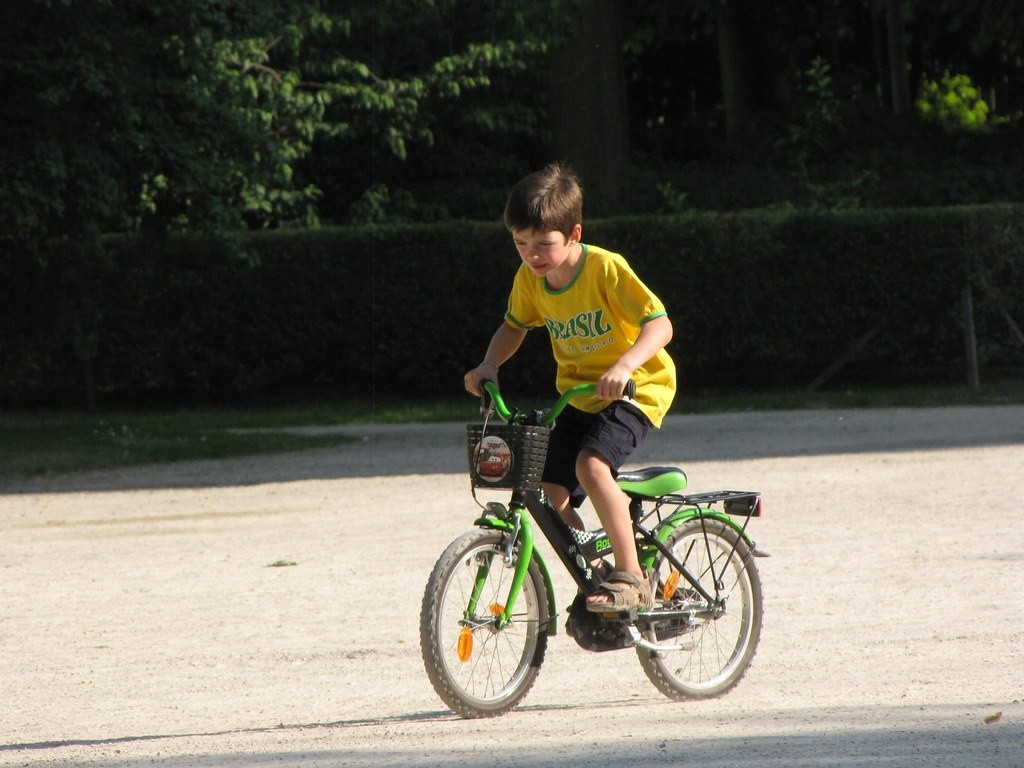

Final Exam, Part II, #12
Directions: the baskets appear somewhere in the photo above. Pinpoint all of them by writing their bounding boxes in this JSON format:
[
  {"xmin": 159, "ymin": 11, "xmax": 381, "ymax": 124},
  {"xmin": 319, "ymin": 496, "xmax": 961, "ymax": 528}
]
[{"xmin": 466, "ymin": 424, "xmax": 550, "ymax": 492}]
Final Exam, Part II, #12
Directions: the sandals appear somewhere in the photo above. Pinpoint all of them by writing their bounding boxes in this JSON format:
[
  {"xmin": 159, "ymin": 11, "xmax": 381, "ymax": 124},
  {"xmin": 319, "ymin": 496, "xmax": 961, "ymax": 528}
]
[{"xmin": 586, "ymin": 571, "xmax": 654, "ymax": 612}]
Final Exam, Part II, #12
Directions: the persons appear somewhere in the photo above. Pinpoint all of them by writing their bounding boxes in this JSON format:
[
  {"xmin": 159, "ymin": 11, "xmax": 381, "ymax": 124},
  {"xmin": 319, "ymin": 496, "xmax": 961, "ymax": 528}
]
[{"xmin": 464, "ymin": 162, "xmax": 677, "ymax": 614}]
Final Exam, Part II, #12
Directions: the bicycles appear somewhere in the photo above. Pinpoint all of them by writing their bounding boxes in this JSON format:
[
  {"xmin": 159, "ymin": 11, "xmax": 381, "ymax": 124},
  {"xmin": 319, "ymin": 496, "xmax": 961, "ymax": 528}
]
[{"xmin": 419, "ymin": 378, "xmax": 776, "ymax": 719}]
